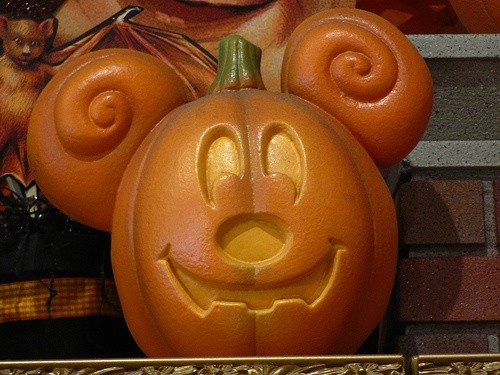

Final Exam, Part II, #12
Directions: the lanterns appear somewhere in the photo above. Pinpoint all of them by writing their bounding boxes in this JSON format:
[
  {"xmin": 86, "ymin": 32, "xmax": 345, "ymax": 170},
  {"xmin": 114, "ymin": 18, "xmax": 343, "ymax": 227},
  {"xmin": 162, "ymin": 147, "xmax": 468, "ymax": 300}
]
[{"xmin": 106, "ymin": 39, "xmax": 399, "ymax": 361}]
